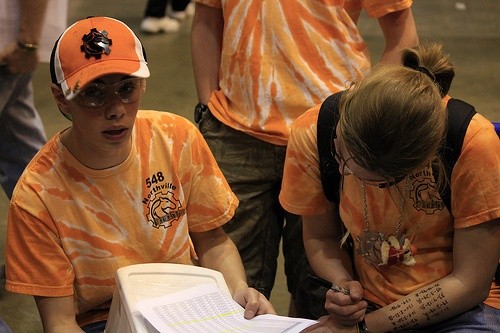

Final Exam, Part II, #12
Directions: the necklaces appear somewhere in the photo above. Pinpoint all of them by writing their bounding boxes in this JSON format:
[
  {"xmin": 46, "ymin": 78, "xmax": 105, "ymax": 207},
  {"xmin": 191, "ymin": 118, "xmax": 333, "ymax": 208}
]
[{"xmin": 358, "ymin": 175, "xmax": 417, "ymax": 267}]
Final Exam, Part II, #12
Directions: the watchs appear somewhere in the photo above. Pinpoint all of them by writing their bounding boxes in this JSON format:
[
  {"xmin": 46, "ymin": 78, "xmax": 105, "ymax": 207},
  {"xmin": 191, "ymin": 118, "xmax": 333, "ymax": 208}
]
[{"xmin": 194, "ymin": 103, "xmax": 209, "ymax": 124}]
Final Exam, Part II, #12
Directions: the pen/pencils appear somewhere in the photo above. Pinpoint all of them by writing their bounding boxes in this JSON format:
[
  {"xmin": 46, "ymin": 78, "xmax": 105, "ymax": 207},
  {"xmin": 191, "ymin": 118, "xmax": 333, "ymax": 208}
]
[{"xmin": 304, "ymin": 272, "xmax": 377, "ymax": 309}]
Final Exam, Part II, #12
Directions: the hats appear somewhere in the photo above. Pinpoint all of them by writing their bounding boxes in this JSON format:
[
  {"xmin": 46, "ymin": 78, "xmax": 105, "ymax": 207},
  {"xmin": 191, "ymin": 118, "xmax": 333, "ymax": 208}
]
[{"xmin": 49, "ymin": 16, "xmax": 150, "ymax": 101}]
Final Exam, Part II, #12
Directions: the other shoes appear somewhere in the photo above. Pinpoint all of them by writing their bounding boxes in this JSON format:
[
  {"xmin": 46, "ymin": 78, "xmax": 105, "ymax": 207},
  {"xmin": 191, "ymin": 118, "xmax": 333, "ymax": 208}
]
[
  {"xmin": 140, "ymin": 15, "xmax": 179, "ymax": 32},
  {"xmin": 170, "ymin": 4, "xmax": 194, "ymax": 19}
]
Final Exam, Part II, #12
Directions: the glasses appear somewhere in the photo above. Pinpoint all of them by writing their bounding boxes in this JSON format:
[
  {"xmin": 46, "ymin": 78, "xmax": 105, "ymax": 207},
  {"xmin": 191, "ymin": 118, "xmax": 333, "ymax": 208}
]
[
  {"xmin": 72, "ymin": 78, "xmax": 146, "ymax": 108},
  {"xmin": 330, "ymin": 127, "xmax": 408, "ymax": 188}
]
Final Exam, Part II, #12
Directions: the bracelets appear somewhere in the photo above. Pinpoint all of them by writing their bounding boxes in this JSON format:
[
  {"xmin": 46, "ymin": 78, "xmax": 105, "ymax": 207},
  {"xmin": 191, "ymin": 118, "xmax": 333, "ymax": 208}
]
[
  {"xmin": 357, "ymin": 319, "xmax": 368, "ymax": 333},
  {"xmin": 17, "ymin": 41, "xmax": 40, "ymax": 50}
]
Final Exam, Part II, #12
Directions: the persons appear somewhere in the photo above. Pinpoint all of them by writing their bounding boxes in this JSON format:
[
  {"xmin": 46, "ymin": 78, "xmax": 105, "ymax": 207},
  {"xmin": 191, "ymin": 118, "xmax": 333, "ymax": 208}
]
[
  {"xmin": 190, "ymin": 0, "xmax": 419, "ymax": 322},
  {"xmin": 5, "ymin": 16, "xmax": 276, "ymax": 333},
  {"xmin": 278, "ymin": 42, "xmax": 500, "ymax": 333},
  {"xmin": 141, "ymin": 0, "xmax": 195, "ymax": 34},
  {"xmin": 0, "ymin": 0, "xmax": 68, "ymax": 201}
]
